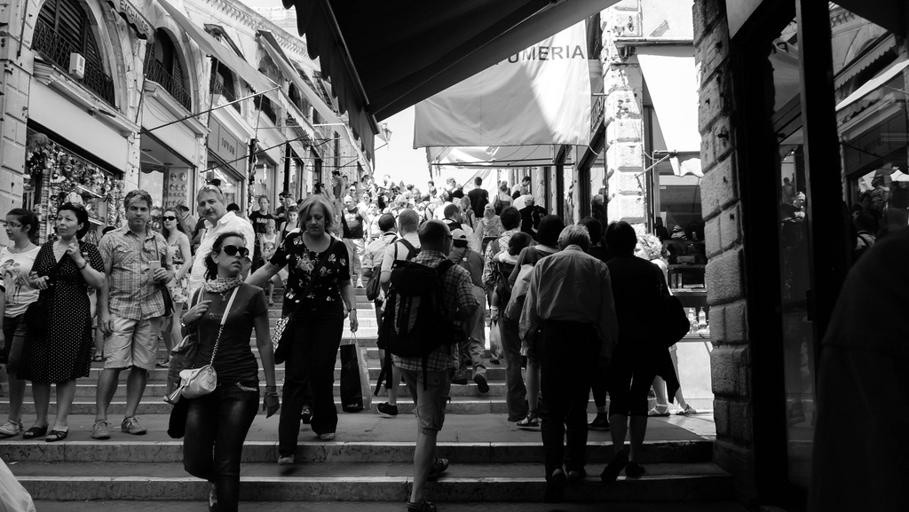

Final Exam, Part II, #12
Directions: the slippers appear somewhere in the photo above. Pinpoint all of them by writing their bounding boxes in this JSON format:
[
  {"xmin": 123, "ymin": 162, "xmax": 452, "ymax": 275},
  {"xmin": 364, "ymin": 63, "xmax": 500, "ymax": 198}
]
[
  {"xmin": 45, "ymin": 424, "xmax": 70, "ymax": 442},
  {"xmin": 23, "ymin": 421, "xmax": 50, "ymax": 439}
]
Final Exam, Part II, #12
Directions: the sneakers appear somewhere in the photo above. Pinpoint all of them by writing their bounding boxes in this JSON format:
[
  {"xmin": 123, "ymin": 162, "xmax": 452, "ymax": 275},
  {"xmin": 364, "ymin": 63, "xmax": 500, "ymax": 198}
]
[
  {"xmin": 0, "ymin": 417, "xmax": 24, "ymax": 436},
  {"xmin": 646, "ymin": 402, "xmax": 691, "ymax": 415},
  {"xmin": 319, "ymin": 431, "xmax": 336, "ymax": 442},
  {"xmin": 600, "ymin": 448, "xmax": 647, "ymax": 484},
  {"xmin": 544, "ymin": 464, "xmax": 586, "ymax": 503},
  {"xmin": 91, "ymin": 415, "xmax": 112, "ymax": 439},
  {"xmin": 587, "ymin": 411, "xmax": 610, "ymax": 431},
  {"xmin": 120, "ymin": 415, "xmax": 148, "ymax": 434},
  {"xmin": 425, "ymin": 454, "xmax": 449, "ymax": 481},
  {"xmin": 473, "ymin": 365, "xmax": 491, "ymax": 393},
  {"xmin": 377, "ymin": 401, "xmax": 399, "ymax": 415},
  {"xmin": 276, "ymin": 452, "xmax": 296, "ymax": 475},
  {"xmin": 406, "ymin": 499, "xmax": 437, "ymax": 511},
  {"xmin": 206, "ymin": 480, "xmax": 217, "ymax": 510},
  {"xmin": 515, "ymin": 414, "xmax": 544, "ymax": 432}
]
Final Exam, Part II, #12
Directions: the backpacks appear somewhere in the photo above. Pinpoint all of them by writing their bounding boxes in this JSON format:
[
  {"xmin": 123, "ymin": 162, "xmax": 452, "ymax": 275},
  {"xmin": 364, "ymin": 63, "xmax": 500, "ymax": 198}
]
[{"xmin": 374, "ymin": 248, "xmax": 465, "ymax": 359}]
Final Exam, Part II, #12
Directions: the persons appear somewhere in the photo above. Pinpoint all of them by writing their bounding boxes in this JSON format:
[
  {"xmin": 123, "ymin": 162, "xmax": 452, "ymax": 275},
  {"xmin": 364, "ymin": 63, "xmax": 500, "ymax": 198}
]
[
  {"xmin": 781, "ymin": 169, "xmax": 909, "ymax": 511},
  {"xmin": 1, "ymin": 168, "xmax": 710, "ymax": 511}
]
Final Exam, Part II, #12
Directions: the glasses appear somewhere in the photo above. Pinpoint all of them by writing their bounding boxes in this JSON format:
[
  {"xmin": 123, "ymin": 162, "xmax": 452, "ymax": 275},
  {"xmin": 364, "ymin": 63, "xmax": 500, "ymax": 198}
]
[
  {"xmin": 162, "ymin": 215, "xmax": 177, "ymax": 222},
  {"xmin": 3, "ymin": 223, "xmax": 23, "ymax": 228},
  {"xmin": 222, "ymin": 244, "xmax": 250, "ymax": 257}
]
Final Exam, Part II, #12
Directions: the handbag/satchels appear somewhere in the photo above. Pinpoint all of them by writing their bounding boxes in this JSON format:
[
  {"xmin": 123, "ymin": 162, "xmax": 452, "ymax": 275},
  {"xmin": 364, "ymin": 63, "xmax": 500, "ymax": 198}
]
[
  {"xmin": 490, "ymin": 262, "xmax": 512, "ymax": 308},
  {"xmin": 24, "ymin": 301, "xmax": 50, "ymax": 330},
  {"xmin": 503, "ymin": 263, "xmax": 537, "ymax": 322},
  {"xmin": 365, "ymin": 260, "xmax": 383, "ymax": 302},
  {"xmin": 335, "ymin": 327, "xmax": 374, "ymax": 414},
  {"xmin": 267, "ymin": 310, "xmax": 297, "ymax": 364},
  {"xmin": 159, "ymin": 255, "xmax": 176, "ymax": 319},
  {"xmin": 170, "ymin": 361, "xmax": 220, "ymax": 407},
  {"xmin": 658, "ymin": 295, "xmax": 693, "ymax": 349}
]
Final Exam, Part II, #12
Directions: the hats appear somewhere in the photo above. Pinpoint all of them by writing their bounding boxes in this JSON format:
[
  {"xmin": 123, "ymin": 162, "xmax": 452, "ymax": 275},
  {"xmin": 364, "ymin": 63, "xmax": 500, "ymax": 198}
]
[{"xmin": 450, "ymin": 228, "xmax": 469, "ymax": 242}]
